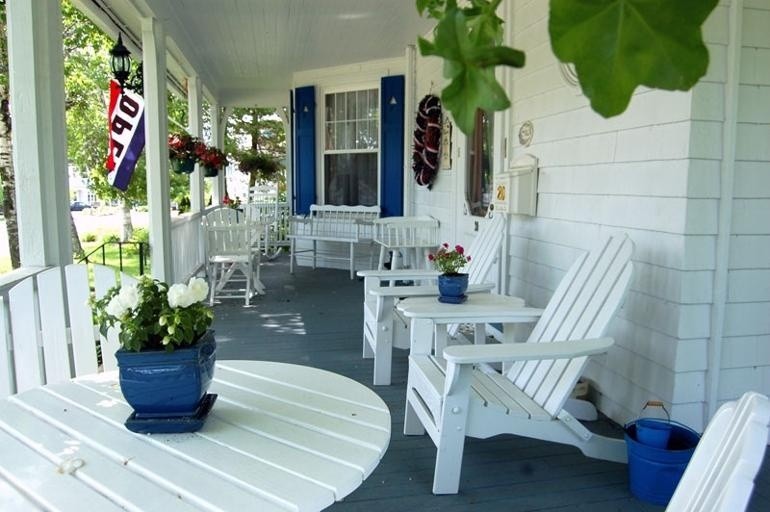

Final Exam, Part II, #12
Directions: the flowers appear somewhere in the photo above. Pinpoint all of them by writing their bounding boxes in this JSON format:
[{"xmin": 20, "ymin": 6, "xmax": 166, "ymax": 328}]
[
  {"xmin": 81, "ymin": 267, "xmax": 217, "ymax": 358},
  {"xmin": 427, "ymin": 241, "xmax": 474, "ymax": 277},
  {"xmin": 194, "ymin": 144, "xmax": 230, "ymax": 171},
  {"xmin": 167, "ymin": 130, "xmax": 206, "ymax": 165}
]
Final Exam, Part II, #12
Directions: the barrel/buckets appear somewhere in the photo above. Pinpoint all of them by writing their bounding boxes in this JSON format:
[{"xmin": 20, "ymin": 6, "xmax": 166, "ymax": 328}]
[
  {"xmin": 635, "ymin": 400, "xmax": 674, "ymax": 449},
  {"xmin": 623, "ymin": 418, "xmax": 702, "ymax": 505}
]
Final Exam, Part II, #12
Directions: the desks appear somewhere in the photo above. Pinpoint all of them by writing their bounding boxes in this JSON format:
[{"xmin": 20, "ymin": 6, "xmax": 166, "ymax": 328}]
[
  {"xmin": 199, "ymin": 220, "xmax": 267, "ymax": 297},
  {"xmin": 393, "ymin": 291, "xmax": 530, "ymax": 437},
  {"xmin": 0, "ymin": 356, "xmax": 394, "ymax": 512}
]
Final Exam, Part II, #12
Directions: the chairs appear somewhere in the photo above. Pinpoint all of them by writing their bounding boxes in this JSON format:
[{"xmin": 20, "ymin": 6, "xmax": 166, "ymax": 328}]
[
  {"xmin": 217, "ymin": 203, "xmax": 263, "ymax": 288},
  {"xmin": 659, "ymin": 390, "xmax": 770, "ymax": 512},
  {"xmin": 356, "ymin": 209, "xmax": 507, "ymax": 389},
  {"xmin": 198, "ymin": 207, "xmax": 266, "ymax": 310},
  {"xmin": 0, "ymin": 261, "xmax": 148, "ymax": 405},
  {"xmin": 402, "ymin": 227, "xmax": 645, "ymax": 496}
]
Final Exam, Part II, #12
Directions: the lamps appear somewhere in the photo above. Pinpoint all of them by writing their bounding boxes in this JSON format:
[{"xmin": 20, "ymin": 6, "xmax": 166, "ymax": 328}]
[{"xmin": 107, "ymin": 31, "xmax": 145, "ymax": 102}]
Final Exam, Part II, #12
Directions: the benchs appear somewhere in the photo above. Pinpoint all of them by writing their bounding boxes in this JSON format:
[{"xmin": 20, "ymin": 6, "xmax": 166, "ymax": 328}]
[{"xmin": 284, "ymin": 204, "xmax": 384, "ymax": 281}]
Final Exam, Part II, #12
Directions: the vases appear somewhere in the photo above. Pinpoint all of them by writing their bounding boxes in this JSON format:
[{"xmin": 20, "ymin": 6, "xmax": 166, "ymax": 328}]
[
  {"xmin": 437, "ymin": 270, "xmax": 469, "ymax": 304},
  {"xmin": 202, "ymin": 165, "xmax": 218, "ymax": 177},
  {"xmin": 112, "ymin": 328, "xmax": 218, "ymax": 435},
  {"xmin": 169, "ymin": 155, "xmax": 194, "ymax": 174}
]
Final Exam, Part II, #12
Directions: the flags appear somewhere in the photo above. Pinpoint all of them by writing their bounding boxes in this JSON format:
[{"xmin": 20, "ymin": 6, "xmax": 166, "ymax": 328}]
[{"xmin": 105, "ymin": 79, "xmax": 145, "ymax": 192}]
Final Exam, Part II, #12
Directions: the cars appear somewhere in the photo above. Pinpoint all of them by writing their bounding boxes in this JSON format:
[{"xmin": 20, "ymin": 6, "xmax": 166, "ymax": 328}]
[{"xmin": 71, "ymin": 202, "xmax": 91, "ymax": 212}]
